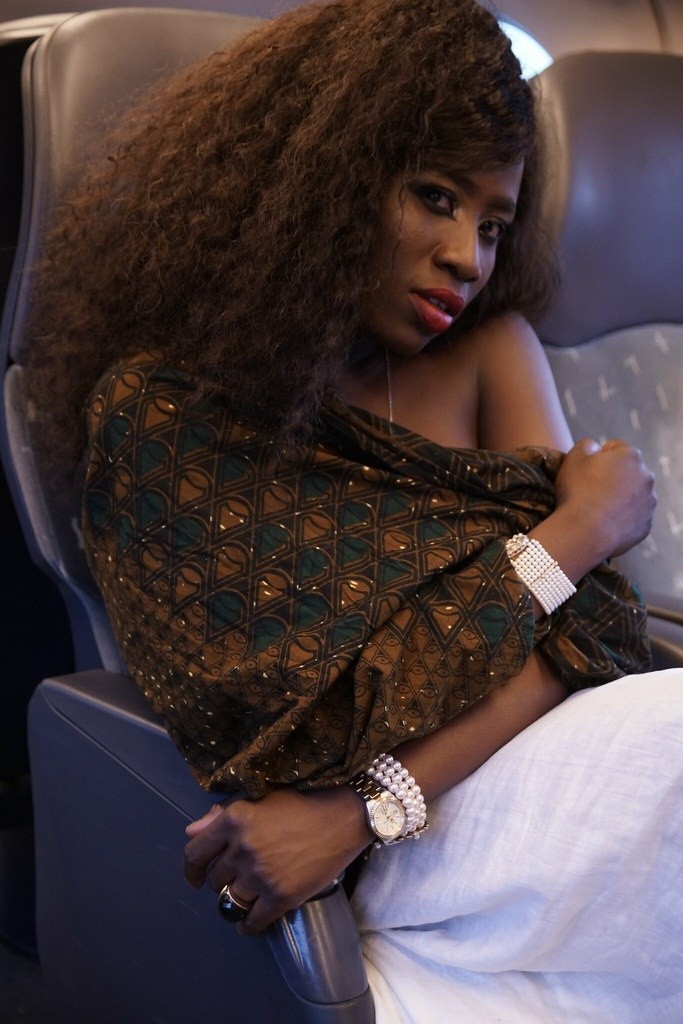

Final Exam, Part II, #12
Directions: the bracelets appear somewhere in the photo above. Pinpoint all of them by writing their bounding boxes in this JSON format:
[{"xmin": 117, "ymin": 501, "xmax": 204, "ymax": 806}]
[
  {"xmin": 506, "ymin": 533, "xmax": 577, "ymax": 615},
  {"xmin": 365, "ymin": 753, "xmax": 430, "ymax": 844}
]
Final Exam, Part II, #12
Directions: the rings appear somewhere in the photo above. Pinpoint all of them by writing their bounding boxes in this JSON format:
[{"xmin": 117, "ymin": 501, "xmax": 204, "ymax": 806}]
[{"xmin": 216, "ymin": 883, "xmax": 252, "ymax": 922}]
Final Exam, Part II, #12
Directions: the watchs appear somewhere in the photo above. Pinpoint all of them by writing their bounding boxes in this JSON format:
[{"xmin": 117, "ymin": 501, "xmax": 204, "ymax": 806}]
[{"xmin": 348, "ymin": 773, "xmax": 407, "ymax": 850}]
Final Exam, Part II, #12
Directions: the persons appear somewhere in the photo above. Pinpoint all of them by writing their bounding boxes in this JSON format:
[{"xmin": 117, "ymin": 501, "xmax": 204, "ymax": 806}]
[{"xmin": 22, "ymin": 0, "xmax": 683, "ymax": 1023}]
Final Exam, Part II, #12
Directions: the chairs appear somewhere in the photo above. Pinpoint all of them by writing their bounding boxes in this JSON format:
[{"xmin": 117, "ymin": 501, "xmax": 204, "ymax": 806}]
[{"xmin": 0, "ymin": 0, "xmax": 683, "ymax": 1024}]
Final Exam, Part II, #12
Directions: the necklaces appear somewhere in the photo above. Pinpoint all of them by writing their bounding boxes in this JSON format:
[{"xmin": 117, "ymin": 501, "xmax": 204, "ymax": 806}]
[{"xmin": 386, "ymin": 351, "xmax": 392, "ymax": 424}]
